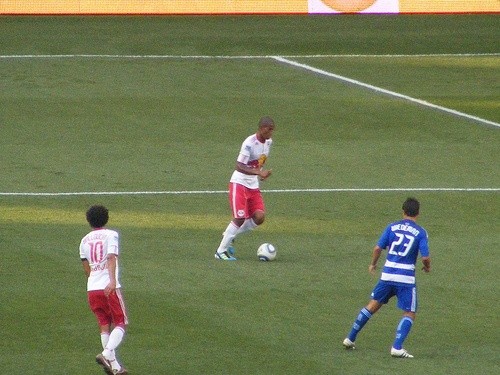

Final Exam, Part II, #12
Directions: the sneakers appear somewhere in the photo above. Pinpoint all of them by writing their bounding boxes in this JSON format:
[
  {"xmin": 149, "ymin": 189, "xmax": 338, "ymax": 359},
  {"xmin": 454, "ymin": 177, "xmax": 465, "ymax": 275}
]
[
  {"xmin": 391, "ymin": 346, "xmax": 414, "ymax": 358},
  {"xmin": 222, "ymin": 232, "xmax": 234, "ymax": 254},
  {"xmin": 214, "ymin": 250, "xmax": 236, "ymax": 261},
  {"xmin": 95, "ymin": 354, "xmax": 127, "ymax": 375},
  {"xmin": 342, "ymin": 338, "xmax": 356, "ymax": 350}
]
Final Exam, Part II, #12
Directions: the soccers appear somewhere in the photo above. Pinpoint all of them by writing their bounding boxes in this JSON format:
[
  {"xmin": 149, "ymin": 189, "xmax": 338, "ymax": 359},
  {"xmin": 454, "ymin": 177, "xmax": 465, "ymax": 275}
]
[{"xmin": 256, "ymin": 243, "xmax": 277, "ymax": 261}]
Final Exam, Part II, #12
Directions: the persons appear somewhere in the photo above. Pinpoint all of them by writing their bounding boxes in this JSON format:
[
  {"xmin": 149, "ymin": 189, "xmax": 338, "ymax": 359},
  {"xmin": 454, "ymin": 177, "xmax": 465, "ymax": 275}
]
[
  {"xmin": 79, "ymin": 205, "xmax": 129, "ymax": 375},
  {"xmin": 215, "ymin": 117, "xmax": 275, "ymax": 260},
  {"xmin": 343, "ymin": 197, "xmax": 430, "ymax": 358}
]
